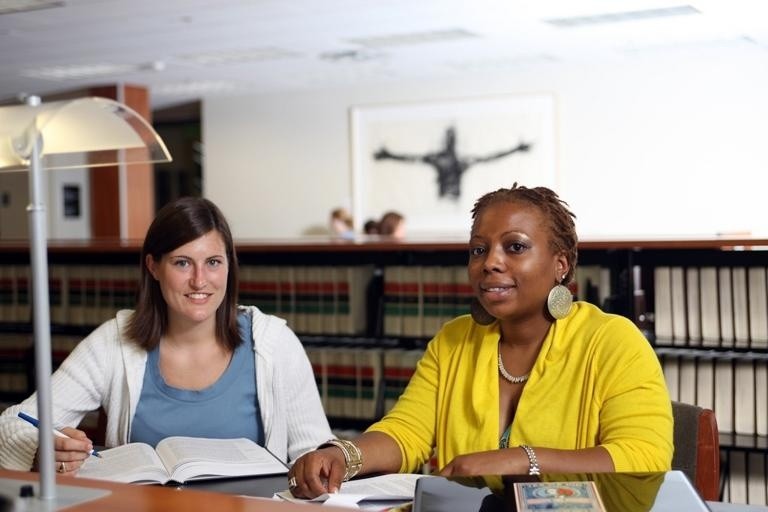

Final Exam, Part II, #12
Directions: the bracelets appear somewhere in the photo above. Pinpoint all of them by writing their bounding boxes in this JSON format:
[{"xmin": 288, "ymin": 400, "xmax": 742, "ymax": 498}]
[{"xmin": 318, "ymin": 438, "xmax": 364, "ymax": 482}]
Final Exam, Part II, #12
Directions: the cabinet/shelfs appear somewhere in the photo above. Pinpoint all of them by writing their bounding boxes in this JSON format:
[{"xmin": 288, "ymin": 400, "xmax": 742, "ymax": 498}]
[{"xmin": 2, "ymin": 239, "xmax": 768, "ymax": 504}]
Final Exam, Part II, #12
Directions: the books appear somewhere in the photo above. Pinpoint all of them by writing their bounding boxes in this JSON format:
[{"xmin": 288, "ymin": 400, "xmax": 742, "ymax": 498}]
[
  {"xmin": 4, "ymin": 254, "xmax": 142, "ymax": 401},
  {"xmin": 52, "ymin": 437, "xmax": 287, "ymax": 484}
]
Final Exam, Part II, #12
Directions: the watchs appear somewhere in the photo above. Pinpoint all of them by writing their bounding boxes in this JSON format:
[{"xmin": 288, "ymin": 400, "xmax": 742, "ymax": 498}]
[{"xmin": 520, "ymin": 443, "xmax": 541, "ymax": 477}]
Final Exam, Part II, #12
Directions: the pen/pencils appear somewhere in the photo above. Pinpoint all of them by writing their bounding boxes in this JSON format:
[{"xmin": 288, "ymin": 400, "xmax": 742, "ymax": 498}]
[{"xmin": 18, "ymin": 412, "xmax": 103, "ymax": 458}]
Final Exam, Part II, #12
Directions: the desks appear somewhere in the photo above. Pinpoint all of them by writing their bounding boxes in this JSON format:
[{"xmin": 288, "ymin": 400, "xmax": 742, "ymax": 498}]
[{"xmin": 0, "ymin": 464, "xmax": 377, "ymax": 511}]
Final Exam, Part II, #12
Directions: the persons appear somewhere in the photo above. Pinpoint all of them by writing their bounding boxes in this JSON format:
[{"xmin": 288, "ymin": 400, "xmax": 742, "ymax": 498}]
[
  {"xmin": 288, "ymin": 187, "xmax": 676, "ymax": 498},
  {"xmin": 1, "ymin": 198, "xmax": 331, "ymax": 473},
  {"xmin": 330, "ymin": 209, "xmax": 405, "ymax": 240}
]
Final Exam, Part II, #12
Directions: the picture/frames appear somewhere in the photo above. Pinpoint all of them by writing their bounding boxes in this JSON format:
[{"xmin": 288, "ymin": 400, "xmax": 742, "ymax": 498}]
[{"xmin": 347, "ymin": 90, "xmax": 565, "ymax": 245}]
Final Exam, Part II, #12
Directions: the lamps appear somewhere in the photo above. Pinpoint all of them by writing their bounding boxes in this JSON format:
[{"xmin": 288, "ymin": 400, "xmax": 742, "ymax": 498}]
[{"xmin": 0, "ymin": 90, "xmax": 172, "ymax": 512}]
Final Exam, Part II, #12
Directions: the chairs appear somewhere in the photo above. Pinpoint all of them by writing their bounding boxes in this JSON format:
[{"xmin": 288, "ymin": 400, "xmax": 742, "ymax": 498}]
[{"xmin": 671, "ymin": 400, "xmax": 721, "ymax": 502}]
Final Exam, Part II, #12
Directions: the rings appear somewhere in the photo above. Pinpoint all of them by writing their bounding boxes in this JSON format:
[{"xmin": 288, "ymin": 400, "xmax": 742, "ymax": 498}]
[
  {"xmin": 58, "ymin": 462, "xmax": 69, "ymax": 472},
  {"xmin": 287, "ymin": 477, "xmax": 294, "ymax": 492}
]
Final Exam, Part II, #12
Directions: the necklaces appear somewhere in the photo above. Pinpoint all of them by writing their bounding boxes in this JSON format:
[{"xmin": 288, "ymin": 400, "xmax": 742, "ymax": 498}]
[{"xmin": 499, "ymin": 344, "xmax": 532, "ymax": 383}]
